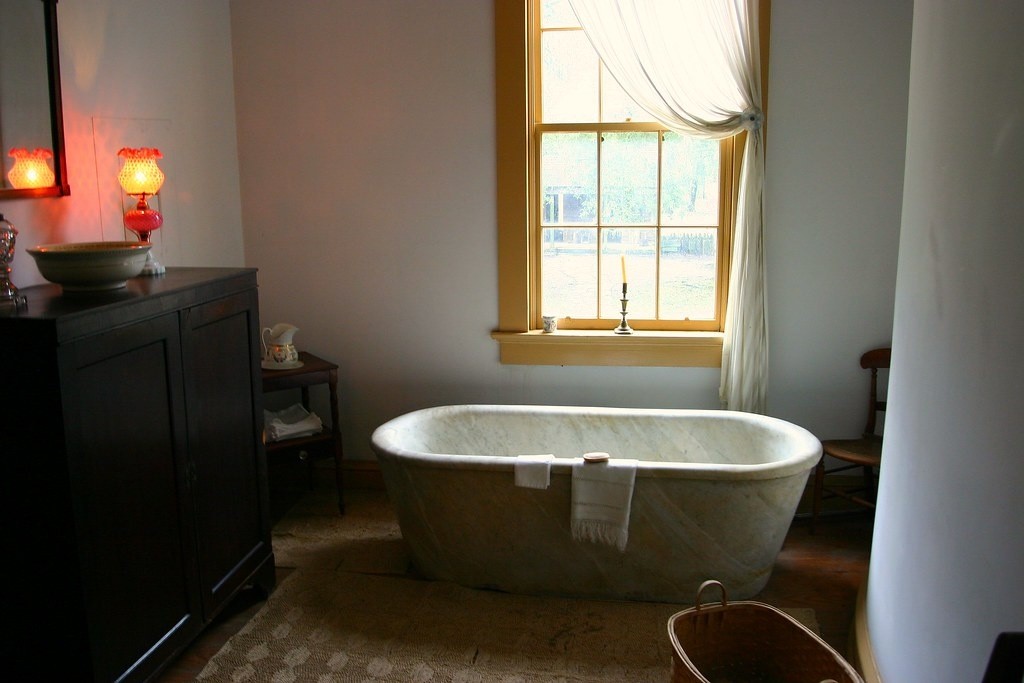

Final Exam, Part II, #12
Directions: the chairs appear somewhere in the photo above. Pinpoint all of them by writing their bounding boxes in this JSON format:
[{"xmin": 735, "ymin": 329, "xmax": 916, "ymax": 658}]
[{"xmin": 811, "ymin": 349, "xmax": 892, "ymax": 535}]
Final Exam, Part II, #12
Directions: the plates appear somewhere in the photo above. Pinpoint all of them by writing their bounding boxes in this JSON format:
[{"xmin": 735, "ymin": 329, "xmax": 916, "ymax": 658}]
[{"xmin": 261, "ymin": 361, "xmax": 303, "ymax": 370}]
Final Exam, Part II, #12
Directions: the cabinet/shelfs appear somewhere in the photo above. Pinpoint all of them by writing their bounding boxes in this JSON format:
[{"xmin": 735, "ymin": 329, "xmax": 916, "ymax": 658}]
[
  {"xmin": 0, "ymin": 266, "xmax": 277, "ymax": 683},
  {"xmin": 261, "ymin": 351, "xmax": 345, "ymax": 515}
]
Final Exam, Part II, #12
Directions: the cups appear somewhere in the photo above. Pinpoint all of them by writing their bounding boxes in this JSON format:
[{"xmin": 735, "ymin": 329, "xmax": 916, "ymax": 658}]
[
  {"xmin": 542, "ymin": 315, "xmax": 557, "ymax": 332},
  {"xmin": 261, "ymin": 322, "xmax": 299, "ymax": 364}
]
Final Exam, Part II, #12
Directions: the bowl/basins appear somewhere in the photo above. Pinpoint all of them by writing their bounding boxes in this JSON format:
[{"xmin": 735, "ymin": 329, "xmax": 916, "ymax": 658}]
[{"xmin": 25, "ymin": 240, "xmax": 153, "ymax": 292}]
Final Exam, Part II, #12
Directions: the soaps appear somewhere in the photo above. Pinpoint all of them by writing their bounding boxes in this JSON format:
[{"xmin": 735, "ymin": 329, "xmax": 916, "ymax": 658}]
[{"xmin": 584, "ymin": 452, "xmax": 608, "ymax": 461}]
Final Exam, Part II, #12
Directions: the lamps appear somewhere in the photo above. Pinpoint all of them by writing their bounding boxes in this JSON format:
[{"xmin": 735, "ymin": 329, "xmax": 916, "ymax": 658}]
[
  {"xmin": 8, "ymin": 147, "xmax": 55, "ymax": 189},
  {"xmin": 117, "ymin": 149, "xmax": 166, "ymax": 277}
]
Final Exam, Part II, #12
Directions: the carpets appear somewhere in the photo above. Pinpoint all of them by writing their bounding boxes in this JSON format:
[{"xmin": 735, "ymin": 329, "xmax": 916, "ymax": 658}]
[
  {"xmin": 196, "ymin": 562, "xmax": 821, "ymax": 683},
  {"xmin": 272, "ymin": 484, "xmax": 408, "ymax": 576}
]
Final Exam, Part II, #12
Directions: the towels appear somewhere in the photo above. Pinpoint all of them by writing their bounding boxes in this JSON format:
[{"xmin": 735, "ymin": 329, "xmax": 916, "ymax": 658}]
[
  {"xmin": 262, "ymin": 403, "xmax": 322, "ymax": 443},
  {"xmin": 514, "ymin": 454, "xmax": 553, "ymax": 488},
  {"xmin": 571, "ymin": 455, "xmax": 636, "ymax": 552}
]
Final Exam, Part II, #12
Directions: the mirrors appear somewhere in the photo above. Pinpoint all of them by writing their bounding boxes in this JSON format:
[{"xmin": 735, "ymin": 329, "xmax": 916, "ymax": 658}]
[{"xmin": 0, "ymin": 0, "xmax": 72, "ymax": 201}]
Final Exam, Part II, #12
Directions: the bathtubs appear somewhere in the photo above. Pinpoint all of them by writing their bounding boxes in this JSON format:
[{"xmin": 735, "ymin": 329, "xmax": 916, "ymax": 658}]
[{"xmin": 371, "ymin": 404, "xmax": 823, "ymax": 604}]
